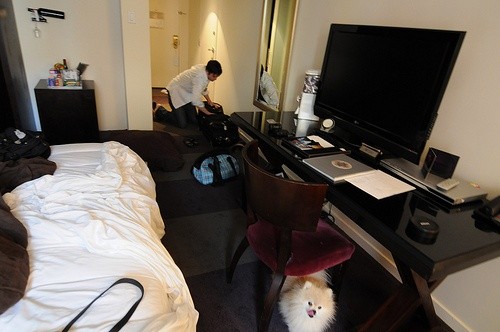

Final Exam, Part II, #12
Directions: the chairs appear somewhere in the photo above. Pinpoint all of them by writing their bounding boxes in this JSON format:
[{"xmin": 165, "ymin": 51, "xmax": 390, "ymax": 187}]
[{"xmin": 227, "ymin": 138, "xmax": 357, "ymax": 332}]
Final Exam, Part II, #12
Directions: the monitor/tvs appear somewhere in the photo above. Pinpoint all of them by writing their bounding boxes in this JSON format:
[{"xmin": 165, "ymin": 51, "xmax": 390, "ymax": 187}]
[{"xmin": 312, "ymin": 23, "xmax": 467, "ymax": 166}]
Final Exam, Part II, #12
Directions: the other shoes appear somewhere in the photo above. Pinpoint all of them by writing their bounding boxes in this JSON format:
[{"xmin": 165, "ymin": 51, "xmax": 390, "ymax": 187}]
[
  {"xmin": 189, "ymin": 137, "xmax": 200, "ymax": 145},
  {"xmin": 182, "ymin": 139, "xmax": 195, "ymax": 148}
]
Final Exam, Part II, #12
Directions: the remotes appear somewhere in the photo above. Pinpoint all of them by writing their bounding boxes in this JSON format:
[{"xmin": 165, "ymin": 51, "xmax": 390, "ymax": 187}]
[{"xmin": 436, "ymin": 177, "xmax": 458, "ymax": 191}]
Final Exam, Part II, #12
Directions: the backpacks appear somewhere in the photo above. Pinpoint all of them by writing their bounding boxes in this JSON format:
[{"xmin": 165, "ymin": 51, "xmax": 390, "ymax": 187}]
[{"xmin": 0, "ymin": 127, "xmax": 51, "ymax": 160}]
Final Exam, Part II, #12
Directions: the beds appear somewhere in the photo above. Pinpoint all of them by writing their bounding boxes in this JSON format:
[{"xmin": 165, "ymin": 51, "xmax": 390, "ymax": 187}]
[{"xmin": 0, "ymin": 141, "xmax": 199, "ymax": 331}]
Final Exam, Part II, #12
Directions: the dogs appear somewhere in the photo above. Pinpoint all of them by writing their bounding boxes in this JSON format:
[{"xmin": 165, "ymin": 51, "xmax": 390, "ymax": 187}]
[{"xmin": 276, "ymin": 270, "xmax": 337, "ymax": 332}]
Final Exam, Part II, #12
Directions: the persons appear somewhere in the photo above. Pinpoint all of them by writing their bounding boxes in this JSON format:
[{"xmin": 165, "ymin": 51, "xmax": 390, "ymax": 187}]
[{"xmin": 152, "ymin": 58, "xmax": 223, "ymax": 130}]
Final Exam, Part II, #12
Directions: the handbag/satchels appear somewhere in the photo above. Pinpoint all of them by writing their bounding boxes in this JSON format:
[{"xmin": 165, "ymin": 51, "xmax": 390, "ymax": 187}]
[
  {"xmin": 198, "ymin": 101, "xmax": 239, "ymax": 145},
  {"xmin": 191, "ymin": 150, "xmax": 240, "ymax": 185}
]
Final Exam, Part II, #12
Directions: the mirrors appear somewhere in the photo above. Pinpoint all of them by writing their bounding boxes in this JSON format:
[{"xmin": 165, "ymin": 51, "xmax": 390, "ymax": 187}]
[{"xmin": 252, "ymin": 0, "xmax": 299, "ymax": 111}]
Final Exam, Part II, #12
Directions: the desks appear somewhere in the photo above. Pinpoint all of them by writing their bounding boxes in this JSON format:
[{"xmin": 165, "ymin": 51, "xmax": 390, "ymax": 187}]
[
  {"xmin": 33, "ymin": 79, "xmax": 102, "ymax": 146},
  {"xmin": 229, "ymin": 109, "xmax": 500, "ymax": 332},
  {"xmin": 429, "ymin": 257, "xmax": 500, "ymax": 332}
]
